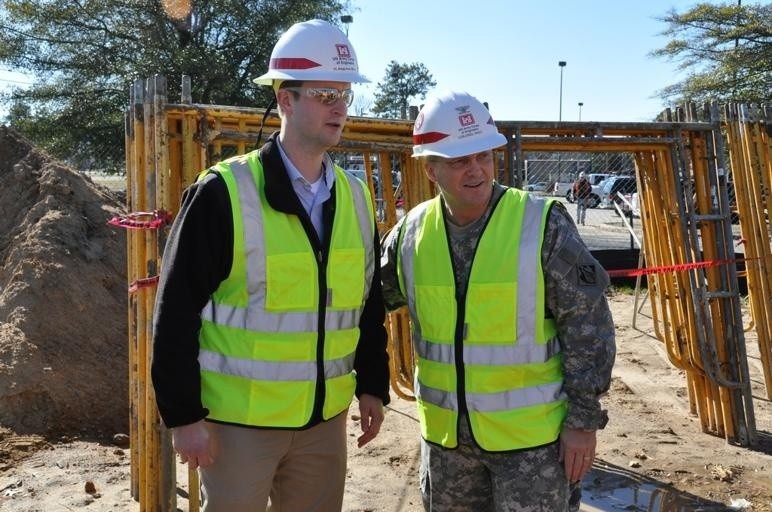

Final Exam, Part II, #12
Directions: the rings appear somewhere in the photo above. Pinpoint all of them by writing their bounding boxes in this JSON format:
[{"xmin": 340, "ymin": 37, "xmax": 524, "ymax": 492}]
[{"xmin": 583, "ymin": 455, "xmax": 592, "ymax": 462}]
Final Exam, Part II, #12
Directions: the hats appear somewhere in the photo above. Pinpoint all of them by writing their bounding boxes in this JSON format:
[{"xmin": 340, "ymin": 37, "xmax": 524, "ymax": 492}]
[{"xmin": 580, "ymin": 172, "xmax": 585, "ymax": 177}]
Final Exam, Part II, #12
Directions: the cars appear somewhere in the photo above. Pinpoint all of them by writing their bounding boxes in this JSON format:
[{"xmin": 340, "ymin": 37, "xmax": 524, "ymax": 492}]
[
  {"xmin": 584, "ymin": 176, "xmax": 634, "ymax": 209},
  {"xmin": 522, "ymin": 182, "xmax": 547, "ymax": 191}
]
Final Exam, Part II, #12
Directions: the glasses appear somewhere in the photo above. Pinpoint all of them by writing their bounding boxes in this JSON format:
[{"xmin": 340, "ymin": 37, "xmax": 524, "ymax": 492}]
[{"xmin": 286, "ymin": 87, "xmax": 353, "ymax": 107}]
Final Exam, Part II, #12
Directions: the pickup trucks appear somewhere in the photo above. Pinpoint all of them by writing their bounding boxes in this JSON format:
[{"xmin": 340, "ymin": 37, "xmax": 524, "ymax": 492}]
[{"xmin": 554, "ymin": 171, "xmax": 615, "ymax": 203}]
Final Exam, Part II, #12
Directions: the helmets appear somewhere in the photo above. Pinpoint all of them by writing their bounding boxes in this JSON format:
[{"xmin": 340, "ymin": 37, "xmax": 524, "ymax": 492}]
[
  {"xmin": 253, "ymin": 18, "xmax": 372, "ymax": 86},
  {"xmin": 411, "ymin": 91, "xmax": 508, "ymax": 158}
]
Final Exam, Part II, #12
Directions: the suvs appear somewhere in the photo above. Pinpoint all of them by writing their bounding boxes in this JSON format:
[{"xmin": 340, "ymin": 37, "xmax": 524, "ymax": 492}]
[{"xmin": 599, "ymin": 175, "xmax": 641, "ymax": 218}]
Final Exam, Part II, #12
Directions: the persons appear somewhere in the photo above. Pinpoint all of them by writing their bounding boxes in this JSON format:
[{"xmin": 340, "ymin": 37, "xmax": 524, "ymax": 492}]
[
  {"xmin": 377, "ymin": 88, "xmax": 616, "ymax": 512},
  {"xmin": 572, "ymin": 170, "xmax": 592, "ymax": 225},
  {"xmin": 150, "ymin": 19, "xmax": 392, "ymax": 512}
]
